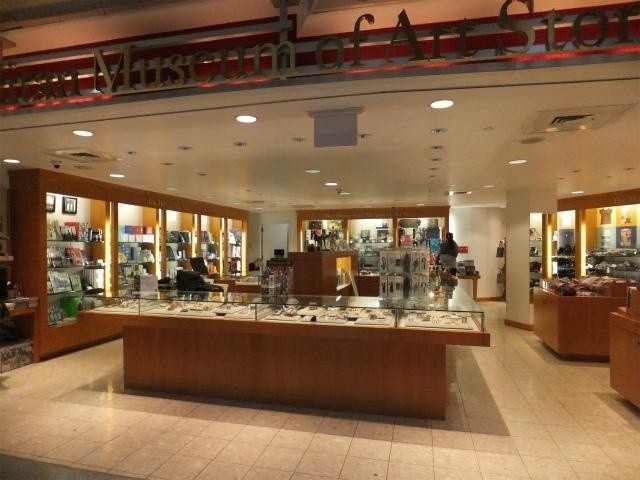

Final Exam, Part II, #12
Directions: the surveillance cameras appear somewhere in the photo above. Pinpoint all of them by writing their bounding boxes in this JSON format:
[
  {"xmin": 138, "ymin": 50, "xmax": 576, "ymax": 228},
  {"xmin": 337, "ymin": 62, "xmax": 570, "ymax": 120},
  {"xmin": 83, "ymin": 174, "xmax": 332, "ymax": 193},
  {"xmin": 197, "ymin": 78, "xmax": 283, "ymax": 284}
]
[{"xmin": 52, "ymin": 161, "xmax": 61, "ymax": 169}]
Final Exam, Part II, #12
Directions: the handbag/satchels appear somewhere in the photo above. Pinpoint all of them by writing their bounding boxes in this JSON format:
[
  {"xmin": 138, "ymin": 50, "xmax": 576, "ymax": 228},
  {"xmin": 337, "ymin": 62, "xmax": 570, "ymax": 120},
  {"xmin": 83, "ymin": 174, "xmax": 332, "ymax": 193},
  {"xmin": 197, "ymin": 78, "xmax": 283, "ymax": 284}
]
[
  {"xmin": 496, "ymin": 247, "xmax": 504, "ymax": 257},
  {"xmin": 497, "ymin": 274, "xmax": 505, "ymax": 284},
  {"xmin": 457, "ymin": 260, "xmax": 476, "ymax": 277},
  {"xmin": 458, "ymin": 247, "xmax": 468, "ymax": 253},
  {"xmin": 530, "ymin": 262, "xmax": 541, "ymax": 272}
]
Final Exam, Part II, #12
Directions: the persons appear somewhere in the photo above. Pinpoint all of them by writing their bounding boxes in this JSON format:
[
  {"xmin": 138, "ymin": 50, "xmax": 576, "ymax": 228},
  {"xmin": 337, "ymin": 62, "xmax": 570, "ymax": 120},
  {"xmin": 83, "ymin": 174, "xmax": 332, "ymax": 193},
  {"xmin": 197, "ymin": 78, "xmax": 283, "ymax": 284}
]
[{"xmin": 435, "ymin": 233, "xmax": 458, "ymax": 276}]
